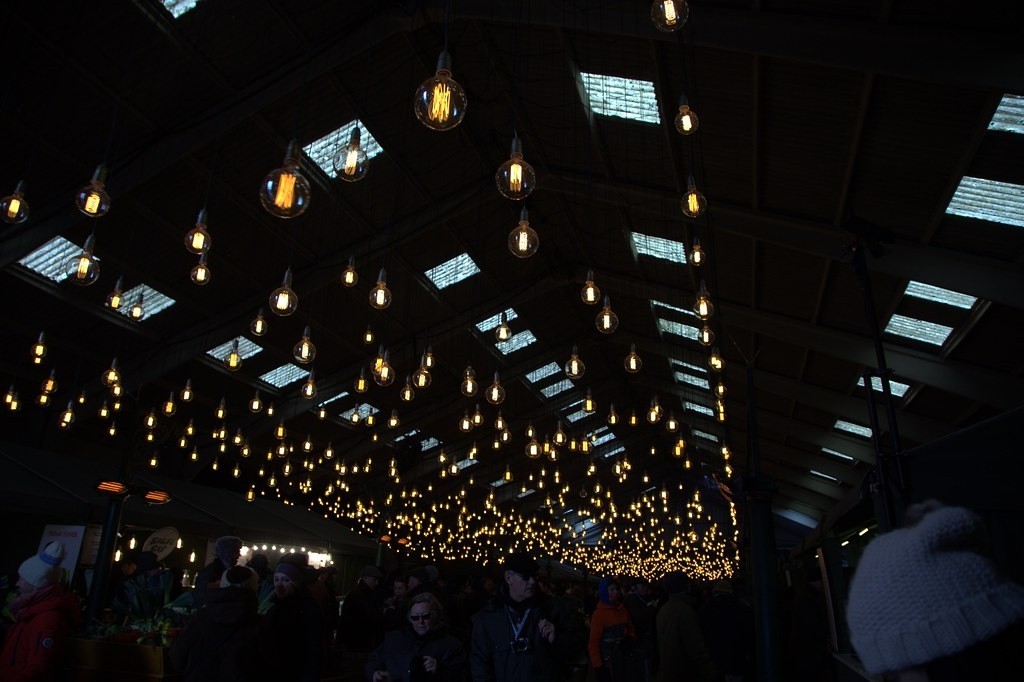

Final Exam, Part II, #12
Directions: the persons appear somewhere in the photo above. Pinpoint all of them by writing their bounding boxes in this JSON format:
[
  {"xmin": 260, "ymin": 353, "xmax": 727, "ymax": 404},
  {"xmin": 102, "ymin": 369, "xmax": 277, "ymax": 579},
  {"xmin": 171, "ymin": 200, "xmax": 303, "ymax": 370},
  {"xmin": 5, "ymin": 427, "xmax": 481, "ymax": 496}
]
[
  {"xmin": 844, "ymin": 503, "xmax": 1024, "ymax": 682},
  {"xmin": 468, "ymin": 551, "xmax": 574, "ymax": 682},
  {"xmin": 0, "ymin": 536, "xmax": 834, "ymax": 682},
  {"xmin": 367, "ymin": 590, "xmax": 467, "ymax": 682},
  {"xmin": 656, "ymin": 578, "xmax": 728, "ymax": 682}
]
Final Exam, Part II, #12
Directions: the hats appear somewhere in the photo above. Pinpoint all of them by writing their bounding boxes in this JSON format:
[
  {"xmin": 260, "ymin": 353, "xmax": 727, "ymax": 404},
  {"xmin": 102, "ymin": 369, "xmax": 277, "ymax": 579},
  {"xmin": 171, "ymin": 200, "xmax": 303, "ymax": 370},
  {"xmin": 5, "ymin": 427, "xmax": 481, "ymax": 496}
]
[
  {"xmin": 363, "ymin": 566, "xmax": 382, "ymax": 577},
  {"xmin": 507, "ymin": 557, "xmax": 539, "ymax": 575},
  {"xmin": 846, "ymin": 501, "xmax": 1024, "ymax": 674},
  {"xmin": 18, "ymin": 540, "xmax": 65, "ymax": 588},
  {"xmin": 220, "ymin": 565, "xmax": 259, "ymax": 591}
]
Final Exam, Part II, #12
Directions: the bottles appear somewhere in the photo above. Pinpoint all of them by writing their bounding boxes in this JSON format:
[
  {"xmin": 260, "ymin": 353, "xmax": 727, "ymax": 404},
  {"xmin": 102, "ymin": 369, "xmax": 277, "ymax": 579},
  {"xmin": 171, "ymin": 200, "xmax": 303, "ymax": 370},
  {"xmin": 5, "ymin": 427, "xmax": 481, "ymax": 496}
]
[{"xmin": 182, "ymin": 570, "xmax": 193, "ymax": 587}]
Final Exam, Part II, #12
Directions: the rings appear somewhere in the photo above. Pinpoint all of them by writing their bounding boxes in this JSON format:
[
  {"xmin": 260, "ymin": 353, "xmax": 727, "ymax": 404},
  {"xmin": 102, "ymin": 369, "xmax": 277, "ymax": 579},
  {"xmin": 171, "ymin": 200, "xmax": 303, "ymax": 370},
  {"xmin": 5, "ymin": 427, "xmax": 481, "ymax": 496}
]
[
  {"xmin": 430, "ymin": 662, "xmax": 433, "ymax": 666},
  {"xmin": 543, "ymin": 623, "xmax": 547, "ymax": 627}
]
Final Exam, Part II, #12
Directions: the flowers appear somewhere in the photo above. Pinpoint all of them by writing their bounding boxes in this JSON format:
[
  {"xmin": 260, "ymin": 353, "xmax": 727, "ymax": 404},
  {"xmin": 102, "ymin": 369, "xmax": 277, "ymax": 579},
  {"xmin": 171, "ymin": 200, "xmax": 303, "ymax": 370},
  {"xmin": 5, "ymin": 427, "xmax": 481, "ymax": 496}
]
[{"xmin": 75, "ymin": 575, "xmax": 195, "ymax": 646}]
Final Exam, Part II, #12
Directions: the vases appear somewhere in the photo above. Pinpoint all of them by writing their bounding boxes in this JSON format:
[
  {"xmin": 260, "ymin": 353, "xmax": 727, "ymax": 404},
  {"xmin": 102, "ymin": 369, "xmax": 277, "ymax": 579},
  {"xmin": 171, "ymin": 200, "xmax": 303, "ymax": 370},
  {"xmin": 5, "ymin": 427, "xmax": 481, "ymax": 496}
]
[{"xmin": 61, "ymin": 635, "xmax": 181, "ymax": 682}]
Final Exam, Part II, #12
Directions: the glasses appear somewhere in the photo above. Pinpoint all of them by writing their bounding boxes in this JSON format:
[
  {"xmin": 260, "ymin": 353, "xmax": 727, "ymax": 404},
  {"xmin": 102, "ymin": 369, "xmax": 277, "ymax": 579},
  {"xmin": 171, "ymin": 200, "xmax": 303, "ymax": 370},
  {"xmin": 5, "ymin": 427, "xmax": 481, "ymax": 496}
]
[
  {"xmin": 513, "ymin": 573, "xmax": 538, "ymax": 581},
  {"xmin": 410, "ymin": 613, "xmax": 432, "ymax": 621}
]
